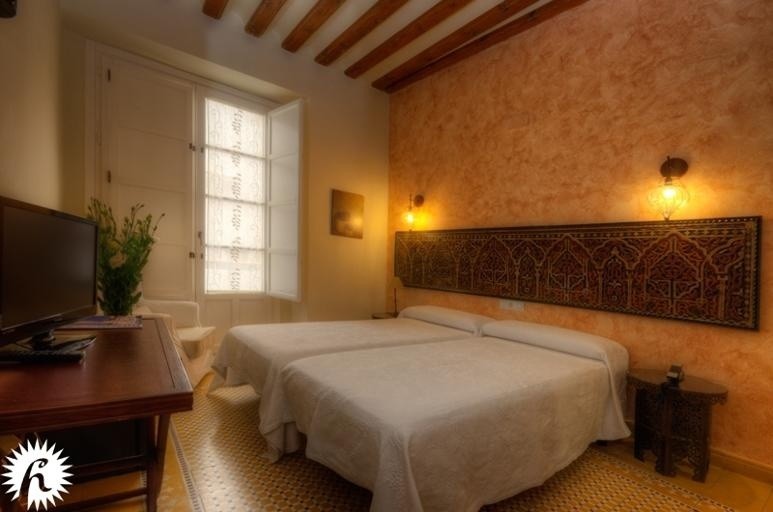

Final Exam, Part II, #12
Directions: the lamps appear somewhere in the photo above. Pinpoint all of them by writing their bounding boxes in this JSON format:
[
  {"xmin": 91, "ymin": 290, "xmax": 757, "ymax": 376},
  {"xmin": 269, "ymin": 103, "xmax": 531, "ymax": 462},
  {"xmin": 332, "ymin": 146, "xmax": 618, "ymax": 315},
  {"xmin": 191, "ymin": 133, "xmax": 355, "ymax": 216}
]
[
  {"xmin": 646, "ymin": 154, "xmax": 689, "ymax": 221},
  {"xmin": 387, "ymin": 276, "xmax": 404, "ymax": 317},
  {"xmin": 402, "ymin": 190, "xmax": 423, "ymax": 226}
]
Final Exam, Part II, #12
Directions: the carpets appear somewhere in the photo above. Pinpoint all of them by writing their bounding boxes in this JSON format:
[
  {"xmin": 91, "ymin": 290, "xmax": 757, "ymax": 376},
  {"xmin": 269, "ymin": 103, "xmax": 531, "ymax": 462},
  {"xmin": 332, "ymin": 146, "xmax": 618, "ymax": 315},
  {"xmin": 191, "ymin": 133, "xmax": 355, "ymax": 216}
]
[{"xmin": 131, "ymin": 381, "xmax": 741, "ymax": 512}]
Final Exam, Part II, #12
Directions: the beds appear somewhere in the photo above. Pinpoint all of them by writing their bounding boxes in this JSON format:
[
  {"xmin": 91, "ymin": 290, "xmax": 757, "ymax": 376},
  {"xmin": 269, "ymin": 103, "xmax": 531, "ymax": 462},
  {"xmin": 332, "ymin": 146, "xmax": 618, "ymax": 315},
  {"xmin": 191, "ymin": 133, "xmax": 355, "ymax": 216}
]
[
  {"xmin": 257, "ymin": 317, "xmax": 635, "ymax": 511},
  {"xmin": 208, "ymin": 299, "xmax": 496, "ymax": 470}
]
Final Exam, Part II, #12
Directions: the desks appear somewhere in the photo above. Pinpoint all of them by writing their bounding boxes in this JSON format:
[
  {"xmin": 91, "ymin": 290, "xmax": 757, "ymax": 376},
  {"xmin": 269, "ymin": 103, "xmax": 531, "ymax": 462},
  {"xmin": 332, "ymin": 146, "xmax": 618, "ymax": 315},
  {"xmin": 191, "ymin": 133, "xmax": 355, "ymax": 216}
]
[
  {"xmin": 370, "ymin": 312, "xmax": 399, "ymax": 320},
  {"xmin": 622, "ymin": 369, "xmax": 731, "ymax": 483}
]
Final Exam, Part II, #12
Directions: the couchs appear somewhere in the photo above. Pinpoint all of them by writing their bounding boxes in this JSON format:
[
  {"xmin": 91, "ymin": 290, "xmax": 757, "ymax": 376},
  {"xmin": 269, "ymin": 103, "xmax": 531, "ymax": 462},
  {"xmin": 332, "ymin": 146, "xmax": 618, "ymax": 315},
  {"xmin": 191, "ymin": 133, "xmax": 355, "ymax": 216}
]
[{"xmin": 94, "ymin": 269, "xmax": 216, "ymax": 395}]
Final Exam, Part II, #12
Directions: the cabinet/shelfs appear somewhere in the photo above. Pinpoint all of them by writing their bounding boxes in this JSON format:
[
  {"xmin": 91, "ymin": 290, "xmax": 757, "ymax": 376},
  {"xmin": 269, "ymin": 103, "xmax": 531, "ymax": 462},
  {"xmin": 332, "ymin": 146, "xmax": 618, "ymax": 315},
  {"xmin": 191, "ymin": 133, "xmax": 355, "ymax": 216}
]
[{"xmin": 0, "ymin": 312, "xmax": 195, "ymax": 512}]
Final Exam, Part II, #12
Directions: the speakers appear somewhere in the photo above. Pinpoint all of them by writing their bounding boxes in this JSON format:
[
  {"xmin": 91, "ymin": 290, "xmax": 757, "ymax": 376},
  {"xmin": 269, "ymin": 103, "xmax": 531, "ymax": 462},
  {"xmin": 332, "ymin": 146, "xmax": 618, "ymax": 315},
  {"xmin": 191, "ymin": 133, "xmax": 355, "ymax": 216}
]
[{"xmin": 0, "ymin": 0, "xmax": 17, "ymax": 19}]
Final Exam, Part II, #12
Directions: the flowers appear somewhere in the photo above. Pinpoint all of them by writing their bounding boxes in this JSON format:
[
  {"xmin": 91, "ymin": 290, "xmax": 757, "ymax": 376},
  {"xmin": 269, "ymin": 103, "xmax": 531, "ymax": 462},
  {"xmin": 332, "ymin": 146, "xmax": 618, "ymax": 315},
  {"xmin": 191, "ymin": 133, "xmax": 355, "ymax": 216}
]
[{"xmin": 83, "ymin": 192, "xmax": 166, "ymax": 317}]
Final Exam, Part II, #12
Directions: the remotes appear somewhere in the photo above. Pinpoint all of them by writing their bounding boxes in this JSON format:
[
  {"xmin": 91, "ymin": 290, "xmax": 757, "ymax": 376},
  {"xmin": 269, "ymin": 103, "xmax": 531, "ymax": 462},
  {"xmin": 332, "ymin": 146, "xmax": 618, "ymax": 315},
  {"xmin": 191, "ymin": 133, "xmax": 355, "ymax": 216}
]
[{"xmin": 0, "ymin": 350, "xmax": 85, "ymax": 362}]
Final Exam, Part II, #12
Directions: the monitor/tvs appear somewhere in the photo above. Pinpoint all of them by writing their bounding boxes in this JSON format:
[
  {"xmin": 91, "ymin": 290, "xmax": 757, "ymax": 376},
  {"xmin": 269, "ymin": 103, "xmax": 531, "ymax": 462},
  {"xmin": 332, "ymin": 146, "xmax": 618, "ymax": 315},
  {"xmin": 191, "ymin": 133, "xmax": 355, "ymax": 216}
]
[{"xmin": 0, "ymin": 195, "xmax": 99, "ymax": 352}]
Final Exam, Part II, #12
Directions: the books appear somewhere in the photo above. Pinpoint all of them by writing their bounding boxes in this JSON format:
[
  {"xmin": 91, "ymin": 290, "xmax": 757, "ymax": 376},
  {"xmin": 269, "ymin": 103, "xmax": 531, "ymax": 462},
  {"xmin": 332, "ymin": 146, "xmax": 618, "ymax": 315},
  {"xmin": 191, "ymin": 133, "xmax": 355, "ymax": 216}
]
[{"xmin": 54, "ymin": 314, "xmax": 143, "ymax": 330}]
